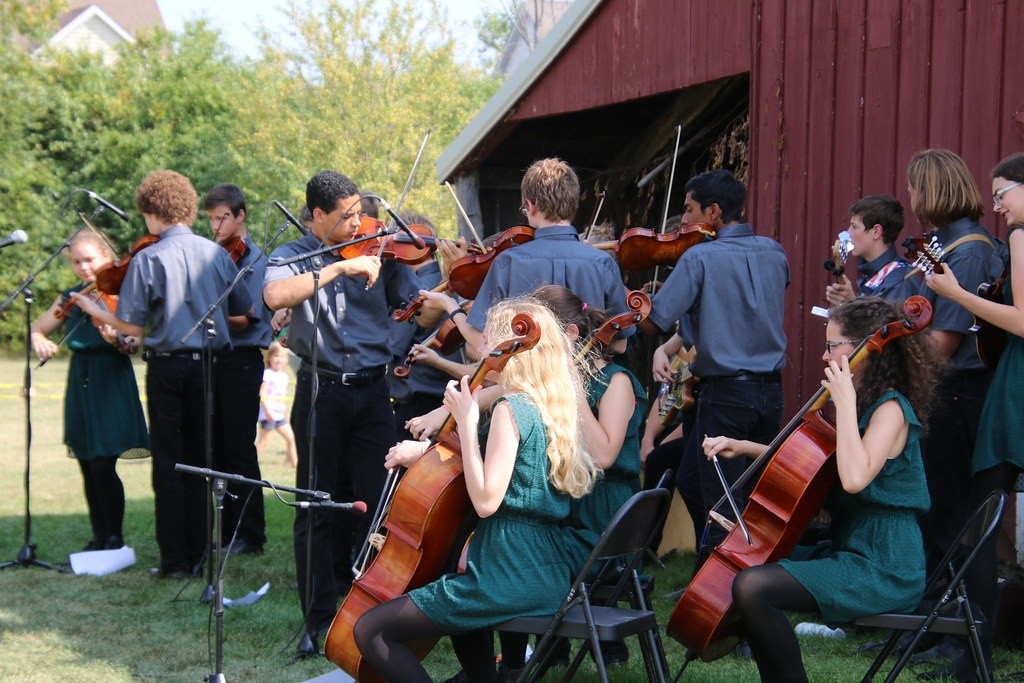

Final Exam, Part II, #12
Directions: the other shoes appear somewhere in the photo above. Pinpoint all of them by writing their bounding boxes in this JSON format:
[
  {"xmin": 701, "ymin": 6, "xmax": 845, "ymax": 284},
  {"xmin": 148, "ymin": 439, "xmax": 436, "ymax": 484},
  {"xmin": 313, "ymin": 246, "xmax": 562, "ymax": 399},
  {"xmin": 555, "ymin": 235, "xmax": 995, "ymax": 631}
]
[
  {"xmin": 908, "ymin": 642, "xmax": 965, "ymax": 667},
  {"xmin": 285, "ymin": 652, "xmax": 321, "ymax": 671},
  {"xmin": 149, "ymin": 567, "xmax": 186, "ymax": 581},
  {"xmin": 497, "ymin": 662, "xmax": 525, "ymax": 683},
  {"xmin": 917, "ymin": 652, "xmax": 995, "ymax": 683}
]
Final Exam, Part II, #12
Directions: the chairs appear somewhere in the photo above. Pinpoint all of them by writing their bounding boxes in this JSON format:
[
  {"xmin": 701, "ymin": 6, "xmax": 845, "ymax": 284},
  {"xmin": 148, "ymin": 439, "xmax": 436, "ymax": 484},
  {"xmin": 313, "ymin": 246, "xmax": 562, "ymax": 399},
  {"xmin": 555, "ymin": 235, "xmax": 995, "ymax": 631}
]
[
  {"xmin": 490, "ymin": 434, "xmax": 691, "ymax": 682},
  {"xmin": 852, "ymin": 489, "xmax": 1011, "ymax": 682}
]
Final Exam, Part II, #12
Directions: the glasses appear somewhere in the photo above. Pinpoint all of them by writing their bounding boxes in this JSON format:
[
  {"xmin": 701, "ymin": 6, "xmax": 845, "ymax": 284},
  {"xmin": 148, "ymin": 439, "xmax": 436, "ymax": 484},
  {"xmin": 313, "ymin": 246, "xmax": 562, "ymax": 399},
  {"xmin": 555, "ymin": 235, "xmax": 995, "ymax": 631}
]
[
  {"xmin": 990, "ymin": 182, "xmax": 1023, "ymax": 206},
  {"xmin": 519, "ymin": 203, "xmax": 527, "ymax": 217},
  {"xmin": 825, "ymin": 339, "xmax": 860, "ymax": 354}
]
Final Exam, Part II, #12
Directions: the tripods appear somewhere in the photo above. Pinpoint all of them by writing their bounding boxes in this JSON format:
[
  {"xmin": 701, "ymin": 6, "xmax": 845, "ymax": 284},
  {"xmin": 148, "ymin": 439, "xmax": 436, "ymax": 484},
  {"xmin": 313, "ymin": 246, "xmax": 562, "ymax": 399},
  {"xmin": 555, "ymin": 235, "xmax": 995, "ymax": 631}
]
[
  {"xmin": 266, "ymin": 225, "xmax": 401, "ymax": 670},
  {"xmin": 0, "ymin": 205, "xmax": 106, "ymax": 573}
]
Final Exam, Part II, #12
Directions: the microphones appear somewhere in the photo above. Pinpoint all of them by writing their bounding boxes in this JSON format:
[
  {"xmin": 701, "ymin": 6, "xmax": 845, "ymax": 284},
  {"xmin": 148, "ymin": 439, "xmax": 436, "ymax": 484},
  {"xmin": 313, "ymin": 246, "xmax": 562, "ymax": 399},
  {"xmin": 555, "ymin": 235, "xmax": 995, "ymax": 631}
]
[
  {"xmin": 290, "ymin": 500, "xmax": 368, "ymax": 515},
  {"xmin": 380, "ymin": 198, "xmax": 425, "ymax": 250},
  {"xmin": 85, "ymin": 190, "xmax": 129, "ymax": 221},
  {"xmin": 273, "ymin": 201, "xmax": 309, "ymax": 237},
  {"xmin": 0, "ymin": 229, "xmax": 27, "ymax": 248}
]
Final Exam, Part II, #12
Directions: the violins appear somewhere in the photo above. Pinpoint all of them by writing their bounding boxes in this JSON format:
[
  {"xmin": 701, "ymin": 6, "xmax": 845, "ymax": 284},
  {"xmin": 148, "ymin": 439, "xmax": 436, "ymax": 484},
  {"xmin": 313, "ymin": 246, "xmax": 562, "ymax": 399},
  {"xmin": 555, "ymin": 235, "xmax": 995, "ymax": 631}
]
[
  {"xmin": 336, "ymin": 213, "xmax": 493, "ymax": 265},
  {"xmin": 588, "ymin": 222, "xmax": 717, "ymax": 271},
  {"xmin": 388, "ymin": 226, "xmax": 535, "ymax": 324},
  {"xmin": 217, "ymin": 236, "xmax": 247, "ymax": 263},
  {"xmin": 392, "ymin": 300, "xmax": 475, "ymax": 378},
  {"xmin": 90, "ymin": 279, "xmax": 139, "ymax": 354},
  {"xmin": 54, "ymin": 235, "xmax": 160, "ymax": 319}
]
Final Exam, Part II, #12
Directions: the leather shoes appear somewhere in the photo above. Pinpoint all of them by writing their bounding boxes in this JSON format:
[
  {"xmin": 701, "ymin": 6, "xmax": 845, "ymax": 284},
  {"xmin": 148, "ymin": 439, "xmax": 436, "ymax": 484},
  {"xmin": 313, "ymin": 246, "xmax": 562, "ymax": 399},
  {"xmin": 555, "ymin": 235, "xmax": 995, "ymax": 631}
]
[{"xmin": 212, "ymin": 536, "xmax": 264, "ymax": 555}]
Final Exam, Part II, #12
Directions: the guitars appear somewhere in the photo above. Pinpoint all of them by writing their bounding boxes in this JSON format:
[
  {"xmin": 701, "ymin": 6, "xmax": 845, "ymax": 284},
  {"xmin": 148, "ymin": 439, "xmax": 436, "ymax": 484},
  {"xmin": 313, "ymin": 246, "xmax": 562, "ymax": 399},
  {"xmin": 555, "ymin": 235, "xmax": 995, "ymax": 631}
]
[
  {"xmin": 658, "ymin": 230, "xmax": 764, "ymax": 425},
  {"xmin": 903, "ymin": 231, "xmax": 1012, "ymax": 372},
  {"xmin": 823, "ymin": 230, "xmax": 855, "ymax": 286}
]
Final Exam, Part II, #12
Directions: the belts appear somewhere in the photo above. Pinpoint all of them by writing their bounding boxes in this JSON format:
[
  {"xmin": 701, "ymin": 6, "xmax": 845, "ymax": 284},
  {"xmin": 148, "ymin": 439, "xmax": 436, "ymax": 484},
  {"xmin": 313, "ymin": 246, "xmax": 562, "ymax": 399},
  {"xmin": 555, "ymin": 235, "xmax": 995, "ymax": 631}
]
[
  {"xmin": 699, "ymin": 370, "xmax": 782, "ymax": 385},
  {"xmin": 301, "ymin": 360, "xmax": 386, "ymax": 385},
  {"xmin": 142, "ymin": 349, "xmax": 218, "ymax": 363}
]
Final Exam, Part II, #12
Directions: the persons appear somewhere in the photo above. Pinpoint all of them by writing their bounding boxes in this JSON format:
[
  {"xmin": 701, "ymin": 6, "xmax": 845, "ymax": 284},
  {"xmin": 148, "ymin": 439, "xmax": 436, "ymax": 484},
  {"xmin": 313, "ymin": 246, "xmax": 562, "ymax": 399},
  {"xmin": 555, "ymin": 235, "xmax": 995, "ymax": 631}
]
[
  {"xmin": 916, "ymin": 153, "xmax": 1024, "ymax": 683},
  {"xmin": 262, "ymin": 157, "xmax": 792, "ymax": 683},
  {"xmin": 701, "ymin": 297, "xmax": 940, "ymax": 683},
  {"xmin": 31, "ymin": 230, "xmax": 152, "ymax": 553},
  {"xmin": 832, "ymin": 191, "xmax": 923, "ymax": 324},
  {"xmin": 197, "ymin": 184, "xmax": 281, "ymax": 559},
  {"xmin": 886, "ymin": 147, "xmax": 1003, "ymax": 682},
  {"xmin": 69, "ymin": 169, "xmax": 253, "ymax": 584}
]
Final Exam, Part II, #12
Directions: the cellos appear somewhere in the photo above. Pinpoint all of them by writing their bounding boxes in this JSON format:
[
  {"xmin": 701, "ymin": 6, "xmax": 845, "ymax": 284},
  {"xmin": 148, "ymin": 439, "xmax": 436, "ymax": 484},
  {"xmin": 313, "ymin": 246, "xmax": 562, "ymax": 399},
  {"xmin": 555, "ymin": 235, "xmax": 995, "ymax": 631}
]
[
  {"xmin": 458, "ymin": 290, "xmax": 652, "ymax": 575},
  {"xmin": 664, "ymin": 294, "xmax": 933, "ymax": 662},
  {"xmin": 323, "ymin": 311, "xmax": 542, "ymax": 682}
]
